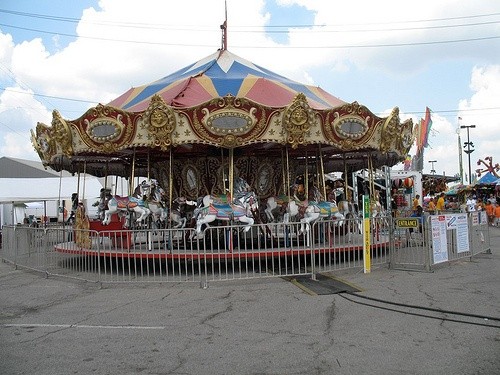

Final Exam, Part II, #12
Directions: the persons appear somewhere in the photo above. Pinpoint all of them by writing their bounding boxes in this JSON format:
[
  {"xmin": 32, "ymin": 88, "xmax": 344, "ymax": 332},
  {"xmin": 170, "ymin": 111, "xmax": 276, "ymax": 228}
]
[{"xmin": 278, "ymin": 174, "xmax": 499, "ymax": 232}]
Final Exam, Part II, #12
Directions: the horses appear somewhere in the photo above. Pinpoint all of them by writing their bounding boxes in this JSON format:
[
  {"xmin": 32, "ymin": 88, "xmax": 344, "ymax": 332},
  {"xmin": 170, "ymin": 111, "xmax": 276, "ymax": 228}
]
[
  {"xmin": 185, "ymin": 177, "xmax": 259, "ymax": 241},
  {"xmin": 259, "ymin": 183, "xmax": 389, "ymax": 236},
  {"xmin": 91, "ymin": 177, "xmax": 188, "ymax": 232}
]
